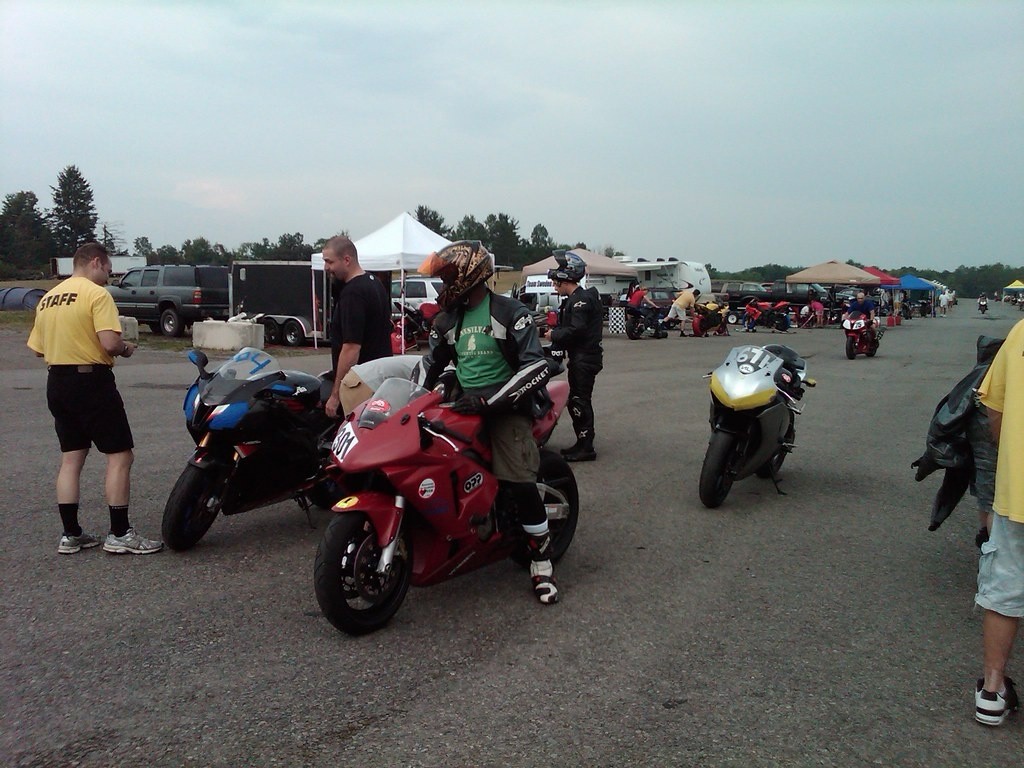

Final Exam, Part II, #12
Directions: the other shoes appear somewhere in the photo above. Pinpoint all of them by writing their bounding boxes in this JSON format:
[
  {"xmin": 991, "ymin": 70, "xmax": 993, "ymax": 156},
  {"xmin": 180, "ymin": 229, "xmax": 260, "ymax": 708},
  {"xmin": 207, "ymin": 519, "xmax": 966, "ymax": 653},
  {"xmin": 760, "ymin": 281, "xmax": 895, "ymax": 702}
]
[
  {"xmin": 680, "ymin": 333, "xmax": 688, "ymax": 337},
  {"xmin": 985, "ymin": 307, "xmax": 989, "ymax": 310},
  {"xmin": 978, "ymin": 308, "xmax": 980, "ymax": 310},
  {"xmin": 660, "ymin": 320, "xmax": 666, "ymax": 327},
  {"xmin": 974, "ymin": 527, "xmax": 989, "ymax": 552}
]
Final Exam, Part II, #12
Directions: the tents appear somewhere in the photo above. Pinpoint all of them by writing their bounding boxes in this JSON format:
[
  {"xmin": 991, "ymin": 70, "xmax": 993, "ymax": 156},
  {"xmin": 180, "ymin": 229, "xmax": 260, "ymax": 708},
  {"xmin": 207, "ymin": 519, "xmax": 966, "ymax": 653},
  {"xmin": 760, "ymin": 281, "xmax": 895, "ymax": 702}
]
[
  {"xmin": 863, "ymin": 266, "xmax": 901, "ymax": 312},
  {"xmin": 1003, "ymin": 280, "xmax": 1024, "ymax": 302},
  {"xmin": 312, "ymin": 213, "xmax": 495, "ymax": 354},
  {"xmin": 518, "ymin": 248, "xmax": 639, "ymax": 301},
  {"xmin": 881, "ymin": 274, "xmax": 936, "ymax": 318},
  {"xmin": 786, "ymin": 261, "xmax": 881, "ymax": 302}
]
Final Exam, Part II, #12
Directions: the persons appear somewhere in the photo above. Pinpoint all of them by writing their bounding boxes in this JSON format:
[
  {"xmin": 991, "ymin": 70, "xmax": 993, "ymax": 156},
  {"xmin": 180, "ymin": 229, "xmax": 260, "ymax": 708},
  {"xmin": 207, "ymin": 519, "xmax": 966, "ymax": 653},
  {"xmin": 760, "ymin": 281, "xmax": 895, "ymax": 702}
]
[
  {"xmin": 321, "ymin": 236, "xmax": 393, "ymax": 416},
  {"xmin": 658, "ymin": 289, "xmax": 700, "ymax": 337},
  {"xmin": 626, "ymin": 288, "xmax": 660, "ymax": 319},
  {"xmin": 545, "ymin": 250, "xmax": 604, "ymax": 461},
  {"xmin": 28, "ymin": 243, "xmax": 163, "ymax": 553},
  {"xmin": 977, "ymin": 292, "xmax": 988, "ymax": 310},
  {"xmin": 939, "ymin": 290, "xmax": 953, "ymax": 318},
  {"xmin": 842, "ymin": 293, "xmax": 878, "ymax": 341},
  {"xmin": 800, "ymin": 299, "xmax": 824, "ymax": 328},
  {"xmin": 995, "ymin": 291, "xmax": 998, "ymax": 301},
  {"xmin": 881, "ymin": 289, "xmax": 907, "ymax": 316},
  {"xmin": 975, "ymin": 319, "xmax": 1024, "ymax": 725},
  {"xmin": 409, "ymin": 241, "xmax": 558, "ymax": 603}
]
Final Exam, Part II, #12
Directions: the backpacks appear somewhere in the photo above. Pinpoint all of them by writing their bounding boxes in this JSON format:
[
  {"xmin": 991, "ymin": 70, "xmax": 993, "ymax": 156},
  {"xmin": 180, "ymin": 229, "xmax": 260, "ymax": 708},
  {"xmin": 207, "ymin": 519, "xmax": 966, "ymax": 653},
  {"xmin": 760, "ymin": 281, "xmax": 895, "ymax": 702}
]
[{"xmin": 927, "ymin": 363, "xmax": 990, "ymax": 467}]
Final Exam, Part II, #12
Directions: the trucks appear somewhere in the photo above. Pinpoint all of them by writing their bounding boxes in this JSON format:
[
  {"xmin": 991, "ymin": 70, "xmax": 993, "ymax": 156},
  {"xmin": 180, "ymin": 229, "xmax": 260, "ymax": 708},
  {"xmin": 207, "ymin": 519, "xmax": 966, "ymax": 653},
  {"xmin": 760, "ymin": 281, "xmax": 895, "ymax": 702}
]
[
  {"xmin": 505, "ymin": 251, "xmax": 714, "ymax": 329},
  {"xmin": 312, "ymin": 374, "xmax": 581, "ymax": 637}
]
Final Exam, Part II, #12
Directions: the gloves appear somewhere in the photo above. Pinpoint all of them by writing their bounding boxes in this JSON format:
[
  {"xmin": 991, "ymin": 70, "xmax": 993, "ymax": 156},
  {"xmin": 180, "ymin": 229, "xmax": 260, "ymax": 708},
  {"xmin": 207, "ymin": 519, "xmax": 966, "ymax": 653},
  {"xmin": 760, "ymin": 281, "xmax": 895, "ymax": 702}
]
[
  {"xmin": 868, "ymin": 320, "xmax": 873, "ymax": 325},
  {"xmin": 449, "ymin": 395, "xmax": 488, "ymax": 415}
]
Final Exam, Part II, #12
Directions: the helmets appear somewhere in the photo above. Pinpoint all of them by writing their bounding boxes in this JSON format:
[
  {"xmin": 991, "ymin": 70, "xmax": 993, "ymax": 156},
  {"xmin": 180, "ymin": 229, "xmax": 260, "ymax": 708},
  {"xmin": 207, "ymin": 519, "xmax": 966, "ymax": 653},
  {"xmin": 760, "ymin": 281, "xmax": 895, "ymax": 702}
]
[
  {"xmin": 429, "ymin": 239, "xmax": 493, "ymax": 308},
  {"xmin": 548, "ymin": 251, "xmax": 586, "ymax": 283},
  {"xmin": 981, "ymin": 292, "xmax": 986, "ymax": 296}
]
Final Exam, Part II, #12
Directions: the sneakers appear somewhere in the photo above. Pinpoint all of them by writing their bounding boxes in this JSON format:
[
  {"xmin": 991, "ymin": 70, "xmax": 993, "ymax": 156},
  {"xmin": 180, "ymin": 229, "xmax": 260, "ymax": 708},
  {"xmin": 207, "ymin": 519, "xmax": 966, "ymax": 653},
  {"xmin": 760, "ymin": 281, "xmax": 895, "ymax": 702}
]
[
  {"xmin": 975, "ymin": 673, "xmax": 1019, "ymax": 726},
  {"xmin": 103, "ymin": 527, "xmax": 163, "ymax": 554},
  {"xmin": 57, "ymin": 530, "xmax": 102, "ymax": 553}
]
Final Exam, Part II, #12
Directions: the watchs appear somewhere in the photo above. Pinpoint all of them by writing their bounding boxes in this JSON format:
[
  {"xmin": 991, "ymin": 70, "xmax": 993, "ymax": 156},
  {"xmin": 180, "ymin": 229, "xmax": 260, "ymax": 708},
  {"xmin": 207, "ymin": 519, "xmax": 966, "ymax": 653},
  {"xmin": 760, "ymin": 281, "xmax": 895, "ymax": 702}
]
[{"xmin": 120, "ymin": 343, "xmax": 129, "ymax": 355}]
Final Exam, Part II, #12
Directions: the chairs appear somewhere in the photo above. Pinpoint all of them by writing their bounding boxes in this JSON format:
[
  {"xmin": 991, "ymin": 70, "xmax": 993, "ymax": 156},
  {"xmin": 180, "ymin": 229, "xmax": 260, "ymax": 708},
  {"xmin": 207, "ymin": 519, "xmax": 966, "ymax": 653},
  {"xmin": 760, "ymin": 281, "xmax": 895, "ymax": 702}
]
[{"xmin": 791, "ymin": 305, "xmax": 814, "ymax": 328}]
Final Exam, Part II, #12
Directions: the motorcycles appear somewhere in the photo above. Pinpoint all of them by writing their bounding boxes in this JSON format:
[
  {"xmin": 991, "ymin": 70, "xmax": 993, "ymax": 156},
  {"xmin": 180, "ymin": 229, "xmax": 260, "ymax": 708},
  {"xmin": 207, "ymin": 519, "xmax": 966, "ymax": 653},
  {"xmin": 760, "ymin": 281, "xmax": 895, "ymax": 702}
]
[
  {"xmin": 690, "ymin": 299, "xmax": 731, "ymax": 337},
  {"xmin": 160, "ymin": 345, "xmax": 371, "ymax": 553},
  {"xmin": 698, "ymin": 344, "xmax": 818, "ymax": 510},
  {"xmin": 609, "ymin": 298, "xmax": 669, "ymax": 340},
  {"xmin": 977, "ymin": 298, "xmax": 989, "ymax": 314},
  {"xmin": 841, "ymin": 311, "xmax": 888, "ymax": 359},
  {"xmin": 741, "ymin": 298, "xmax": 793, "ymax": 334}
]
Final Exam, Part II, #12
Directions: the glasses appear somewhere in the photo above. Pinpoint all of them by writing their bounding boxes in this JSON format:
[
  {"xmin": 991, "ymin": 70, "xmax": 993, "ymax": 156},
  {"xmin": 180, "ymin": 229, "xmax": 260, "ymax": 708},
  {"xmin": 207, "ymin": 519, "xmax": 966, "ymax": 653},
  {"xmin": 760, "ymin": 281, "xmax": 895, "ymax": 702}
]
[{"xmin": 857, "ymin": 298, "xmax": 864, "ymax": 299}]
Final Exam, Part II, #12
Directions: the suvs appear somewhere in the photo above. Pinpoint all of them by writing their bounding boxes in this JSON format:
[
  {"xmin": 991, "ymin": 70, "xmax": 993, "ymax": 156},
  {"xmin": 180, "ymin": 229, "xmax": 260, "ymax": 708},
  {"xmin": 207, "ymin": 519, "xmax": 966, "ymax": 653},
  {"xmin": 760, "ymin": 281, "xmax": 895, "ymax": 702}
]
[
  {"xmin": 719, "ymin": 278, "xmax": 905, "ymax": 325},
  {"xmin": 391, "ymin": 274, "xmax": 448, "ymax": 321},
  {"xmin": 102, "ymin": 260, "xmax": 230, "ymax": 339}
]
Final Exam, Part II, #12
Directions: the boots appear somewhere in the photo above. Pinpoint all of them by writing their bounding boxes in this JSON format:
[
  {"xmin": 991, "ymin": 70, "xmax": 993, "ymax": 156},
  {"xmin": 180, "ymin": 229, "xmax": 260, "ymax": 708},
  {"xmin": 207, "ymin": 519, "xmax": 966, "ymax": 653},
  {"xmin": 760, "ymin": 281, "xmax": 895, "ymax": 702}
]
[
  {"xmin": 559, "ymin": 404, "xmax": 597, "ymax": 462},
  {"xmin": 521, "ymin": 515, "xmax": 559, "ymax": 605}
]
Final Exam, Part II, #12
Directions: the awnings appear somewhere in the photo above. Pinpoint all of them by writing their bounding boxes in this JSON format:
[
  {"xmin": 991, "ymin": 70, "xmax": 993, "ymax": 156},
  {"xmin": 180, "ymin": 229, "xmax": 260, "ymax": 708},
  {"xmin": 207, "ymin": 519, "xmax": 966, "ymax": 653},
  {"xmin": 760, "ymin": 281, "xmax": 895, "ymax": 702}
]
[{"xmin": 628, "ymin": 263, "xmax": 679, "ymax": 288}]
